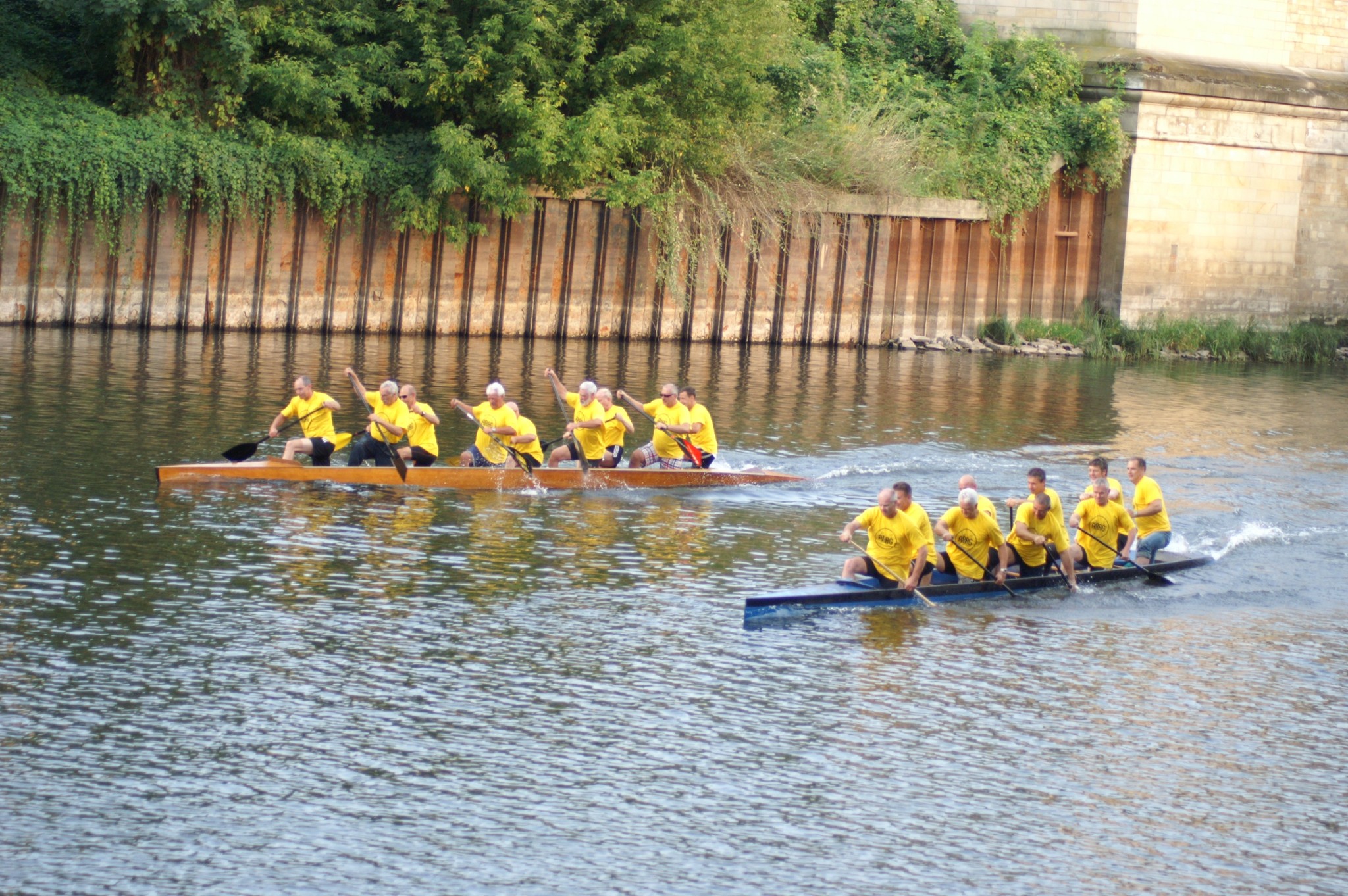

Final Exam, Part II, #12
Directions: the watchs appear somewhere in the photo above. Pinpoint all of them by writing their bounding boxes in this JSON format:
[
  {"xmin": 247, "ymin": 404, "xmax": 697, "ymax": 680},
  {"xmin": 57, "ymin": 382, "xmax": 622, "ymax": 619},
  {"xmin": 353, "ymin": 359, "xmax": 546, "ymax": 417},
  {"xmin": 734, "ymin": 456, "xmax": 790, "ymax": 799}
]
[
  {"xmin": 421, "ymin": 411, "xmax": 425, "ymax": 416},
  {"xmin": 492, "ymin": 427, "xmax": 496, "ymax": 432},
  {"xmin": 1000, "ymin": 568, "xmax": 1008, "ymax": 574},
  {"xmin": 1133, "ymin": 509, "xmax": 1140, "ymax": 517}
]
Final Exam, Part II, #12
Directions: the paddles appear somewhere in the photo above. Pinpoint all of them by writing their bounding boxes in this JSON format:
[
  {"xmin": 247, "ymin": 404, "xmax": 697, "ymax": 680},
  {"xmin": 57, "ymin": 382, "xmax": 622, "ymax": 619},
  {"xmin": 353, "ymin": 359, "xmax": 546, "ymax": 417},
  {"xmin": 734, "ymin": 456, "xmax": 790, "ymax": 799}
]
[
  {"xmin": 547, "ymin": 367, "xmax": 589, "ymax": 476},
  {"xmin": 950, "ymin": 535, "xmax": 1021, "ymax": 598},
  {"xmin": 1076, "ymin": 522, "xmax": 1174, "ymax": 585},
  {"xmin": 221, "ymin": 401, "xmax": 326, "ymax": 463},
  {"xmin": 619, "ymin": 390, "xmax": 702, "ymax": 467},
  {"xmin": 1042, "ymin": 538, "xmax": 1072, "ymax": 589},
  {"xmin": 347, "ymin": 367, "xmax": 409, "ymax": 483},
  {"xmin": 451, "ymin": 397, "xmax": 534, "ymax": 479},
  {"xmin": 539, "ymin": 414, "xmax": 617, "ymax": 453},
  {"xmin": 851, "ymin": 541, "xmax": 936, "ymax": 606},
  {"xmin": 1009, "ymin": 506, "xmax": 1013, "ymax": 531},
  {"xmin": 334, "ymin": 410, "xmax": 413, "ymax": 452}
]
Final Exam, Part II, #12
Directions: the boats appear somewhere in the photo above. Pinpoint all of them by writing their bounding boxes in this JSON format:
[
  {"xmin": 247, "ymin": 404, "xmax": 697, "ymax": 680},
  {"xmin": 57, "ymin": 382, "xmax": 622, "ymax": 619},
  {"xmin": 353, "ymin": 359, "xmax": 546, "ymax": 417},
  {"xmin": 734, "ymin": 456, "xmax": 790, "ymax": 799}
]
[
  {"xmin": 744, "ymin": 552, "xmax": 1207, "ymax": 620},
  {"xmin": 155, "ymin": 464, "xmax": 800, "ymax": 492}
]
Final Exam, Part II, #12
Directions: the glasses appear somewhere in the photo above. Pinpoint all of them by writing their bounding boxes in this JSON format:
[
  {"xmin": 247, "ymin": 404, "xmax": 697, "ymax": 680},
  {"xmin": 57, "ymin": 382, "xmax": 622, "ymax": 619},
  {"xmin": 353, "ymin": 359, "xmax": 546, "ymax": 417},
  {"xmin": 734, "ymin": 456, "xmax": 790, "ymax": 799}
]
[
  {"xmin": 660, "ymin": 394, "xmax": 674, "ymax": 398},
  {"xmin": 399, "ymin": 395, "xmax": 408, "ymax": 399}
]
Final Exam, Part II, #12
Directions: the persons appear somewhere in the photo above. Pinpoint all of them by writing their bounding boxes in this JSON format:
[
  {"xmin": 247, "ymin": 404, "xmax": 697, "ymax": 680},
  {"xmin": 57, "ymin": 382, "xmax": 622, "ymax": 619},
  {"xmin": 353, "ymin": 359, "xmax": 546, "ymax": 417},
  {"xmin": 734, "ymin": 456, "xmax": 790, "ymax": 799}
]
[
  {"xmin": 933, "ymin": 487, "xmax": 1008, "ymax": 587},
  {"xmin": 678, "ymin": 386, "xmax": 718, "ymax": 469},
  {"xmin": 1078, "ymin": 457, "xmax": 1129, "ymax": 552},
  {"xmin": 839, "ymin": 488, "xmax": 928, "ymax": 593},
  {"xmin": 450, "ymin": 382, "xmax": 518, "ymax": 467},
  {"xmin": 1061, "ymin": 477, "xmax": 1137, "ymax": 576},
  {"xmin": 344, "ymin": 367, "xmax": 409, "ymax": 467},
  {"xmin": 616, "ymin": 384, "xmax": 691, "ymax": 470},
  {"xmin": 1123, "ymin": 457, "xmax": 1172, "ymax": 568},
  {"xmin": 954, "ymin": 469, "xmax": 1080, "ymax": 596},
  {"xmin": 562, "ymin": 387, "xmax": 634, "ymax": 469},
  {"xmin": 544, "ymin": 367, "xmax": 605, "ymax": 468},
  {"xmin": 365, "ymin": 384, "xmax": 440, "ymax": 467},
  {"xmin": 505, "ymin": 401, "xmax": 544, "ymax": 468},
  {"xmin": 268, "ymin": 376, "xmax": 341, "ymax": 466},
  {"xmin": 892, "ymin": 481, "xmax": 938, "ymax": 588}
]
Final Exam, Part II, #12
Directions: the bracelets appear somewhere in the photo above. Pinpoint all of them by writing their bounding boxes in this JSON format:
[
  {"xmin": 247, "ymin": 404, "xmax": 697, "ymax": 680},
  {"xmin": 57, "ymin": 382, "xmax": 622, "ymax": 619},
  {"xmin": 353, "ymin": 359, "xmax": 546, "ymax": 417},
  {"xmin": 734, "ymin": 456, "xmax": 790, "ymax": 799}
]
[{"xmin": 666, "ymin": 424, "xmax": 668, "ymax": 429}]
